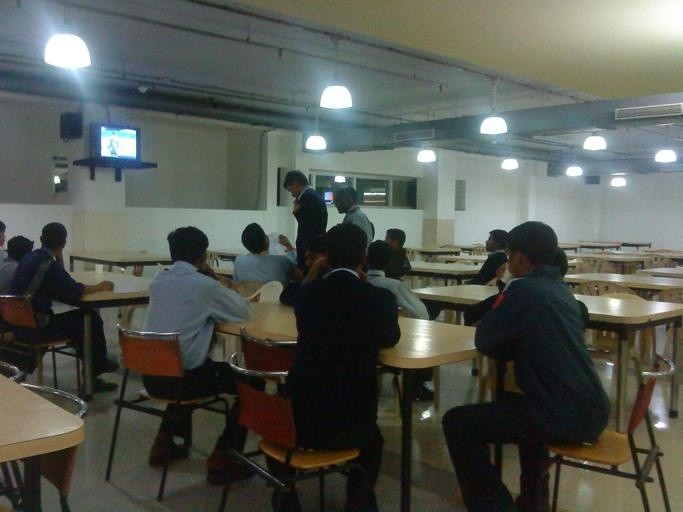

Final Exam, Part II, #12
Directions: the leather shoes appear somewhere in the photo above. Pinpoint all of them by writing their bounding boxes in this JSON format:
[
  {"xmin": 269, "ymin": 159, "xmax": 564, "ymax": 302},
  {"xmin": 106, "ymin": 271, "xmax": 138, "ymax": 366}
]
[
  {"xmin": 210, "ymin": 458, "xmax": 255, "ymax": 484},
  {"xmin": 149, "ymin": 441, "xmax": 191, "ymax": 465}
]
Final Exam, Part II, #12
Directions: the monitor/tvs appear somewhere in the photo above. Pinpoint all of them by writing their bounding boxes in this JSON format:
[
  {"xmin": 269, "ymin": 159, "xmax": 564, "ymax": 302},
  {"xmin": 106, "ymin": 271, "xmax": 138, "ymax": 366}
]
[
  {"xmin": 324, "ymin": 191, "xmax": 334, "ymax": 204},
  {"xmin": 90, "ymin": 122, "xmax": 143, "ymax": 164}
]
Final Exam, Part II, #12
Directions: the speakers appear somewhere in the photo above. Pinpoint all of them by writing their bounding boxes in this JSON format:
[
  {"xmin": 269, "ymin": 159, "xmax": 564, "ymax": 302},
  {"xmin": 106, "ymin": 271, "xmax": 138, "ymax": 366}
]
[{"xmin": 60, "ymin": 113, "xmax": 83, "ymax": 138}]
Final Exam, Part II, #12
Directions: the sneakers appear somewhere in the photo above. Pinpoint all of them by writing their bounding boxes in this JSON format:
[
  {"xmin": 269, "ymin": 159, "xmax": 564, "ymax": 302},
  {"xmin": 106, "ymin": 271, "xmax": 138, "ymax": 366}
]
[
  {"xmin": 83, "ymin": 361, "xmax": 120, "ymax": 376},
  {"xmin": 79, "ymin": 379, "xmax": 118, "ymax": 392}
]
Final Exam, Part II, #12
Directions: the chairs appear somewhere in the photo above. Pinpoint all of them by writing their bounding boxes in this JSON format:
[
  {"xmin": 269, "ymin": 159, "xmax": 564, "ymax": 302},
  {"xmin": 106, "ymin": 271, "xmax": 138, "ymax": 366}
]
[
  {"xmin": 576, "ymin": 282, "xmax": 651, "ymax": 412},
  {"xmin": 471, "ymin": 245, "xmax": 487, "ymax": 255},
  {"xmin": 103, "ymin": 323, "xmax": 230, "ymax": 504},
  {"xmin": 0, "ymin": 361, "xmax": 25, "ymax": 382},
  {"xmin": 41, "ymin": 381, "xmax": 88, "ymax": 512},
  {"xmin": 0, "ymin": 295, "xmax": 85, "ymax": 397},
  {"xmin": 548, "ymin": 352, "xmax": 675, "ymax": 511},
  {"xmin": 218, "ymin": 327, "xmax": 359, "ymax": 512}
]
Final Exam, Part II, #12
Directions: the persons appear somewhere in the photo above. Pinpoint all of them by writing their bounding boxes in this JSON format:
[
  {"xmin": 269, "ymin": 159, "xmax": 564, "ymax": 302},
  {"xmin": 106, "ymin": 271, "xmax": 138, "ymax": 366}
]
[
  {"xmin": 8, "ymin": 223, "xmax": 119, "ymax": 394},
  {"xmin": 331, "ymin": 185, "xmax": 374, "ymax": 278},
  {"xmin": 234, "ymin": 223, "xmax": 298, "ymax": 297},
  {"xmin": 0, "ymin": 223, "xmax": 7, "ymax": 263},
  {"xmin": 443, "ymin": 222, "xmax": 610, "ymax": 512},
  {"xmin": 361, "ymin": 239, "xmax": 435, "ymax": 401},
  {"xmin": 140, "ymin": 227, "xmax": 268, "ymax": 485},
  {"xmin": 384, "ymin": 230, "xmax": 411, "ymax": 274},
  {"xmin": 298, "ymin": 238, "xmax": 331, "ymax": 297},
  {"xmin": 0, "ymin": 235, "xmax": 47, "ymax": 381},
  {"xmin": 271, "ymin": 224, "xmax": 401, "ymax": 511},
  {"xmin": 464, "ymin": 229, "xmax": 506, "ymax": 288},
  {"xmin": 282, "ymin": 170, "xmax": 327, "ymax": 270}
]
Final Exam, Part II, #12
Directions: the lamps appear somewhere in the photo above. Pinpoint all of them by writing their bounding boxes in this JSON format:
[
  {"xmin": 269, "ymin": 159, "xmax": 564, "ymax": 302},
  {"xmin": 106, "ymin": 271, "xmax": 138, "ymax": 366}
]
[
  {"xmin": 565, "ymin": 164, "xmax": 581, "ymax": 176},
  {"xmin": 609, "ymin": 175, "xmax": 626, "ymax": 190},
  {"xmin": 480, "ymin": 85, "xmax": 507, "ymax": 135},
  {"xmin": 582, "ymin": 133, "xmax": 607, "ymax": 154},
  {"xmin": 319, "ymin": 39, "xmax": 354, "ymax": 111},
  {"xmin": 500, "ymin": 155, "xmax": 520, "ymax": 171},
  {"xmin": 44, "ymin": 1, "xmax": 92, "ymax": 71},
  {"xmin": 305, "ymin": 112, "xmax": 326, "ymax": 151},
  {"xmin": 417, "ymin": 147, "xmax": 438, "ymax": 164},
  {"xmin": 653, "ymin": 146, "xmax": 679, "ymax": 166}
]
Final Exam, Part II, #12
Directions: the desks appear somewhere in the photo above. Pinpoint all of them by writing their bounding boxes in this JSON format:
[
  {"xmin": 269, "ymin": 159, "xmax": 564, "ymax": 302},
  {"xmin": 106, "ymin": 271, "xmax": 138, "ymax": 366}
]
[
  {"xmin": 575, "ymin": 293, "xmax": 681, "ymax": 435},
  {"xmin": 219, "ymin": 300, "xmax": 481, "ymax": 512},
  {"xmin": 411, "ymin": 285, "xmax": 498, "ymax": 325},
  {"xmin": 205, "ymin": 249, "xmax": 243, "ymax": 265},
  {"xmin": 65, "ymin": 249, "xmax": 174, "ymax": 273},
  {"xmin": 212, "ymin": 267, "xmax": 235, "ymax": 281},
  {"xmin": 410, "ymin": 262, "xmax": 482, "ymax": 286},
  {"xmin": 573, "ymin": 257, "xmax": 614, "ymax": 273},
  {"xmin": 437, "ymin": 255, "xmax": 488, "ymax": 263},
  {"xmin": 581, "ymin": 242, "xmax": 620, "ymax": 253},
  {"xmin": 416, "ymin": 247, "xmax": 461, "ymax": 260},
  {"xmin": 0, "ymin": 372, "xmax": 87, "ymax": 512},
  {"xmin": 607, "ymin": 255, "xmax": 645, "ymax": 275},
  {"xmin": 564, "ymin": 272, "xmax": 683, "ymax": 298},
  {"xmin": 70, "ymin": 269, "xmax": 152, "ymax": 397},
  {"xmin": 558, "ymin": 243, "xmax": 579, "ymax": 253},
  {"xmin": 635, "ymin": 268, "xmax": 682, "ymax": 278},
  {"xmin": 644, "ymin": 250, "xmax": 683, "ymax": 260}
]
